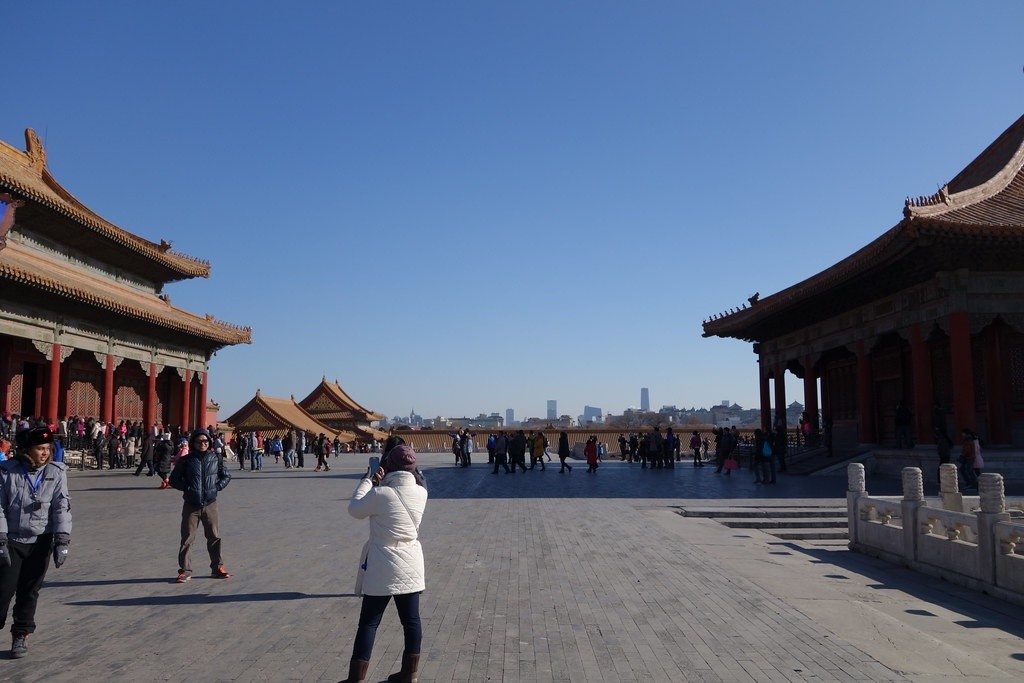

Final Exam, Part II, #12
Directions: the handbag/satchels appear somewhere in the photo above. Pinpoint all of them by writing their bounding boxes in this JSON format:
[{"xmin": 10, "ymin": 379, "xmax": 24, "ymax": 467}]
[
  {"xmin": 724, "ymin": 454, "xmax": 736, "ymax": 468},
  {"xmin": 763, "ymin": 441, "xmax": 772, "ymax": 456}
]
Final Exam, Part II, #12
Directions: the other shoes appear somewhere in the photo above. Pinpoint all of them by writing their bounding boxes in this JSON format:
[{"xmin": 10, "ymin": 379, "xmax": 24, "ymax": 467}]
[
  {"xmin": 159, "ymin": 478, "xmax": 170, "ymax": 489},
  {"xmin": 694, "ymin": 462, "xmax": 704, "ymax": 467},
  {"xmin": 132, "ymin": 473, "xmax": 139, "ymax": 476},
  {"xmin": 714, "ymin": 470, "xmax": 731, "ymax": 475},
  {"xmin": 752, "ymin": 480, "xmax": 776, "ymax": 485},
  {"xmin": 314, "ymin": 466, "xmax": 331, "ymax": 472},
  {"xmin": 586, "ymin": 468, "xmax": 597, "ymax": 473},
  {"xmin": 558, "ymin": 467, "xmax": 572, "ymax": 473}
]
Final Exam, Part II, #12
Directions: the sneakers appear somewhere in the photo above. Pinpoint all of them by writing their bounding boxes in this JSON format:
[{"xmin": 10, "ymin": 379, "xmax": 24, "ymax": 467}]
[
  {"xmin": 11, "ymin": 636, "xmax": 28, "ymax": 658},
  {"xmin": 211, "ymin": 566, "xmax": 230, "ymax": 579},
  {"xmin": 176, "ymin": 574, "xmax": 192, "ymax": 583}
]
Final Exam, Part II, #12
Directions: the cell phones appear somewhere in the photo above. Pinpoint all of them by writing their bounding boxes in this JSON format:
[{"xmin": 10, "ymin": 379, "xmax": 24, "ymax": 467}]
[{"xmin": 369, "ymin": 456, "xmax": 381, "ymax": 476}]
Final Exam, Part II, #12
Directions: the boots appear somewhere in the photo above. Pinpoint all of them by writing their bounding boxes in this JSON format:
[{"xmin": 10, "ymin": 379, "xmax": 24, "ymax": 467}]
[
  {"xmin": 388, "ymin": 650, "xmax": 420, "ymax": 683},
  {"xmin": 337, "ymin": 659, "xmax": 369, "ymax": 683}
]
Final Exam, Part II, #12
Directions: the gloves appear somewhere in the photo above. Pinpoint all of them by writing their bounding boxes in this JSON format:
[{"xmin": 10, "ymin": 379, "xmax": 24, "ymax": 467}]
[
  {"xmin": 361, "ymin": 466, "xmax": 374, "ymax": 482},
  {"xmin": 0, "ymin": 532, "xmax": 11, "ymax": 566},
  {"xmin": 52, "ymin": 545, "xmax": 69, "ymax": 569}
]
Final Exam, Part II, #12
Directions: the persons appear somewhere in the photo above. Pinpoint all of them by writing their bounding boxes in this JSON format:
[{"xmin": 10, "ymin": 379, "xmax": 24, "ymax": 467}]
[
  {"xmin": 892, "ymin": 400, "xmax": 914, "ymax": 449},
  {"xmin": 618, "ymin": 426, "xmax": 680, "ymax": 469},
  {"xmin": 338, "ymin": 445, "xmax": 428, "ymax": 683},
  {"xmin": 0, "ymin": 427, "xmax": 72, "ymax": 657},
  {"xmin": 206, "ymin": 424, "xmax": 386, "ymax": 472},
  {"xmin": 448, "ymin": 426, "xmax": 478, "ymax": 468},
  {"xmin": 0, "ymin": 414, "xmax": 191, "ymax": 489},
  {"xmin": 930, "ymin": 422, "xmax": 985, "ymax": 494},
  {"xmin": 583, "ymin": 435, "xmax": 604, "ymax": 472},
  {"xmin": 689, "ymin": 412, "xmax": 812, "ymax": 484},
  {"xmin": 372, "ymin": 436, "xmax": 427, "ymax": 490},
  {"xmin": 557, "ymin": 432, "xmax": 572, "ymax": 473},
  {"xmin": 168, "ymin": 429, "xmax": 231, "ymax": 583},
  {"xmin": 486, "ymin": 430, "xmax": 552, "ymax": 475}
]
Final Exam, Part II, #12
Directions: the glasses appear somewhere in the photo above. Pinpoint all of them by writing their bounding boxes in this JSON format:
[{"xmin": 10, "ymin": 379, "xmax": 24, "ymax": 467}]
[{"xmin": 194, "ymin": 439, "xmax": 208, "ymax": 443}]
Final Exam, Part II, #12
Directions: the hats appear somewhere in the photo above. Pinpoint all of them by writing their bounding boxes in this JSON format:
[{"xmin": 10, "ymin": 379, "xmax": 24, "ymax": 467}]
[
  {"xmin": 666, "ymin": 427, "xmax": 673, "ymax": 431},
  {"xmin": 386, "ymin": 445, "xmax": 416, "ymax": 471},
  {"xmin": 15, "ymin": 427, "xmax": 53, "ymax": 448}
]
